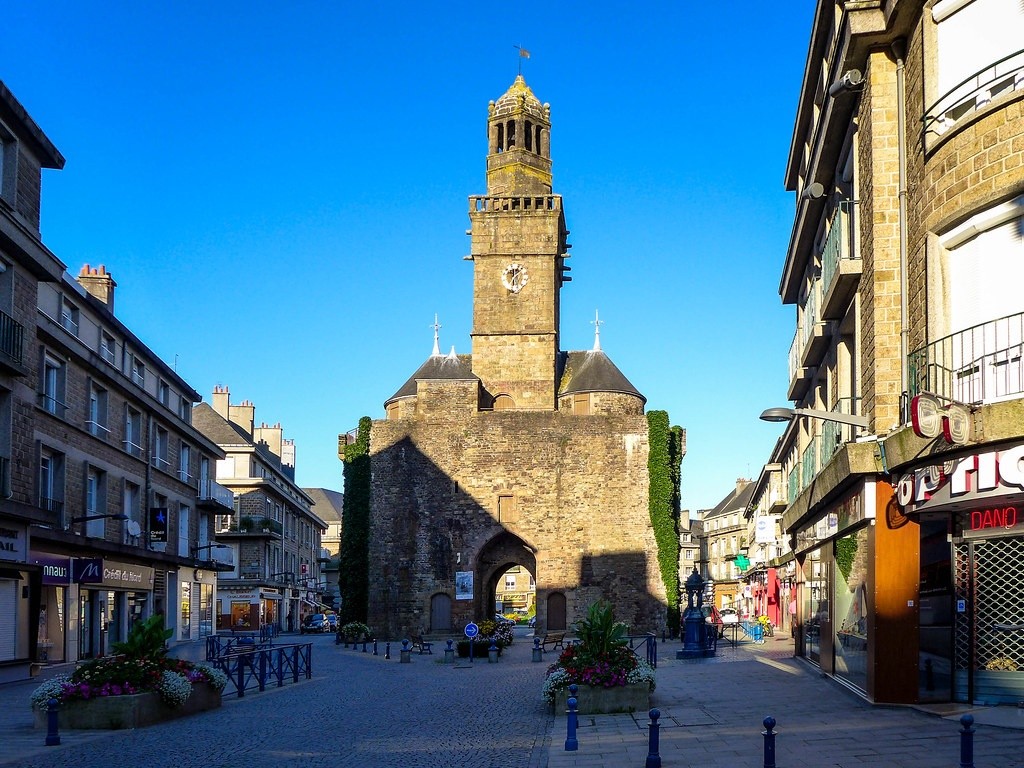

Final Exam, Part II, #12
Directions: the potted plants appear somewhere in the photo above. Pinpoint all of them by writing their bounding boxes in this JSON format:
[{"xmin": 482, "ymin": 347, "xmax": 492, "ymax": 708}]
[
  {"xmin": 259, "ymin": 519, "xmax": 275, "ymax": 533},
  {"xmin": 229, "ymin": 525, "xmax": 237, "ymax": 534},
  {"xmin": 238, "ymin": 516, "xmax": 253, "ymax": 533},
  {"xmin": 341, "ymin": 621, "xmax": 371, "ymax": 644}
]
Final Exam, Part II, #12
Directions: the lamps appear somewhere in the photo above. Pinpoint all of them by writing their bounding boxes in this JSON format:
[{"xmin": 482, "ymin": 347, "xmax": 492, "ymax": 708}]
[
  {"xmin": 315, "ymin": 582, "xmax": 332, "ymax": 585},
  {"xmin": 271, "ymin": 572, "xmax": 296, "ymax": 576},
  {"xmin": 759, "ymin": 408, "xmax": 866, "ymax": 430},
  {"xmin": 298, "ymin": 577, "xmax": 317, "ymax": 582}
]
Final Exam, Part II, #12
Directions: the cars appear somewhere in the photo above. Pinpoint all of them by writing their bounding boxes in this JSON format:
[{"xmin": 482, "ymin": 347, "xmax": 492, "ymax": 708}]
[
  {"xmin": 528, "ymin": 616, "xmax": 536, "ymax": 628},
  {"xmin": 301, "ymin": 613, "xmax": 330, "ymax": 634},
  {"xmin": 496, "ymin": 613, "xmax": 516, "ymax": 626},
  {"xmin": 325, "ymin": 610, "xmax": 340, "ymax": 633}
]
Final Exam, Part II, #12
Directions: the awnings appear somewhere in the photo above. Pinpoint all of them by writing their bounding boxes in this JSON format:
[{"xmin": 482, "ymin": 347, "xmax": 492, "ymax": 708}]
[
  {"xmin": 302, "ymin": 599, "xmax": 331, "ymax": 609},
  {"xmin": 261, "ymin": 593, "xmax": 283, "ymax": 599}
]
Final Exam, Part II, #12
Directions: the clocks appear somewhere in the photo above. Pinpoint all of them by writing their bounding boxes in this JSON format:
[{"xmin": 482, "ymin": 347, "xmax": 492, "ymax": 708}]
[{"xmin": 503, "ymin": 265, "xmax": 528, "ymax": 291}]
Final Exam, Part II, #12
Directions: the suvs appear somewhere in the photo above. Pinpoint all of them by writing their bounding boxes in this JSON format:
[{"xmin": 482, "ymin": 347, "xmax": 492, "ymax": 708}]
[{"xmin": 681, "ymin": 604, "xmax": 724, "ymax": 643}]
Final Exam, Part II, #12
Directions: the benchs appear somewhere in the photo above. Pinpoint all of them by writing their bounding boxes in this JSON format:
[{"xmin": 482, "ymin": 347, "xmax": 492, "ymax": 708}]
[
  {"xmin": 410, "ymin": 634, "xmax": 434, "ymax": 656},
  {"xmin": 540, "ymin": 631, "xmax": 567, "ymax": 654}
]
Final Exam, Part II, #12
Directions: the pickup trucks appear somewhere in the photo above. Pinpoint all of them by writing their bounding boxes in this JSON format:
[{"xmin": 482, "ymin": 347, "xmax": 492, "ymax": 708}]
[{"xmin": 719, "ymin": 608, "xmax": 738, "ymax": 628}]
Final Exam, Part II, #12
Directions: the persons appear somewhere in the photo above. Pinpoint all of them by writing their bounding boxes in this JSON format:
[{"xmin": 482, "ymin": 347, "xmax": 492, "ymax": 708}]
[
  {"xmin": 285, "ymin": 611, "xmax": 296, "ymax": 633},
  {"xmin": 751, "ymin": 613, "xmax": 770, "ymax": 637},
  {"xmin": 265, "ymin": 610, "xmax": 274, "ymax": 638}
]
